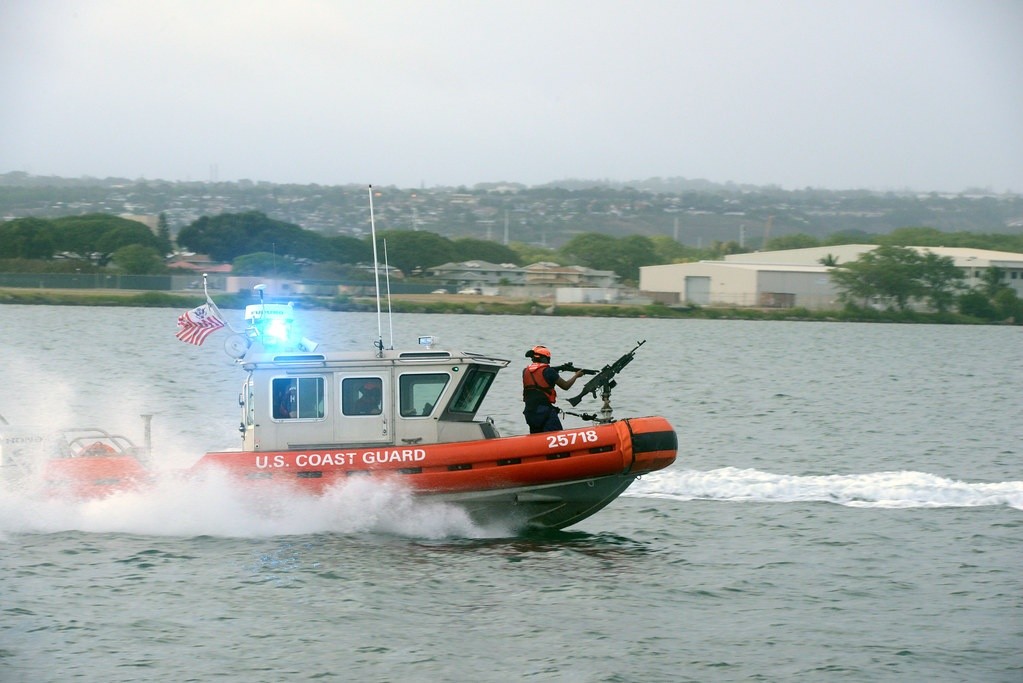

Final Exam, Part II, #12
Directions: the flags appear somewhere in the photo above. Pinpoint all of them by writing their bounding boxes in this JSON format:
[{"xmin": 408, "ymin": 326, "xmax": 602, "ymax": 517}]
[{"xmin": 175, "ymin": 303, "xmax": 224, "ymax": 346}]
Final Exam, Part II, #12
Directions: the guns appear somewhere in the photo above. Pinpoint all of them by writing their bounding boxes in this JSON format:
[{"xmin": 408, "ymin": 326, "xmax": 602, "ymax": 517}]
[
  {"xmin": 566, "ymin": 340, "xmax": 647, "ymax": 407},
  {"xmin": 551, "ymin": 361, "xmax": 600, "ymax": 375}
]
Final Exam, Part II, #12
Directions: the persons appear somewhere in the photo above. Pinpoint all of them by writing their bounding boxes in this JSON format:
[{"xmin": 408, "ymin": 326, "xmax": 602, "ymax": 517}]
[
  {"xmin": 354, "ymin": 389, "xmax": 381, "ymax": 413},
  {"xmin": 522, "ymin": 346, "xmax": 583, "ymax": 434},
  {"xmin": 279, "ymin": 383, "xmax": 297, "ymax": 418}
]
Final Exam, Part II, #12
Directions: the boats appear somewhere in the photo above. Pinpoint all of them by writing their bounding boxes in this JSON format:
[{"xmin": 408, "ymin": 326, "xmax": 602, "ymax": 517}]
[{"xmin": 23, "ymin": 183, "xmax": 679, "ymax": 541}]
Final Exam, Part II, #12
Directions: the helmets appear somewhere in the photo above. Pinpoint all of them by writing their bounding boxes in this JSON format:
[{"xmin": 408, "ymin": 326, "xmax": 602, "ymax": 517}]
[{"xmin": 532, "ymin": 345, "xmax": 550, "ymax": 359}]
